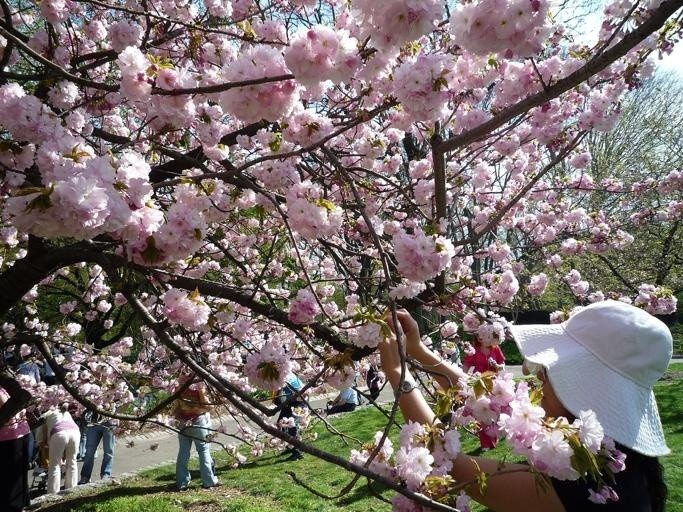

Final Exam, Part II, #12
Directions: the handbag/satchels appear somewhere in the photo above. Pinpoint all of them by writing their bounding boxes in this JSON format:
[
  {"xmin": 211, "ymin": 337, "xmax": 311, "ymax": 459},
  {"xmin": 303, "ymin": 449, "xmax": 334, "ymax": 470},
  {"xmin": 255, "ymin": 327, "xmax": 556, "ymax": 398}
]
[{"xmin": 176, "ymin": 389, "xmax": 208, "ymax": 416}]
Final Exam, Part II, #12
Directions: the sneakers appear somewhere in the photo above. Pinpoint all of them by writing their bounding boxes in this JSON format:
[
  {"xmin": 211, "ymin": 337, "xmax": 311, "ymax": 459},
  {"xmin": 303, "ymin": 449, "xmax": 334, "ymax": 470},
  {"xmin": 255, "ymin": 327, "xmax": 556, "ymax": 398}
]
[{"xmin": 80, "ymin": 478, "xmax": 92, "ymax": 484}]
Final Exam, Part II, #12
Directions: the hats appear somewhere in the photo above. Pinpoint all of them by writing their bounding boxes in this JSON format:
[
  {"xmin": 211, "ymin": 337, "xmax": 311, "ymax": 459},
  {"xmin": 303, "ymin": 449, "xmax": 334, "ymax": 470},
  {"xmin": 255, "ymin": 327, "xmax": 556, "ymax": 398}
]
[{"xmin": 507, "ymin": 301, "xmax": 673, "ymax": 458}]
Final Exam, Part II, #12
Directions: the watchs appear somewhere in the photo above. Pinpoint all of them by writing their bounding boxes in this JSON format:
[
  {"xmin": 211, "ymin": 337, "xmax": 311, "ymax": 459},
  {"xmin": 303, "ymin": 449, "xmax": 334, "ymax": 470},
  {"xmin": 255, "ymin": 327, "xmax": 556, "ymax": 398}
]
[{"xmin": 398, "ymin": 380, "xmax": 419, "ymax": 392}]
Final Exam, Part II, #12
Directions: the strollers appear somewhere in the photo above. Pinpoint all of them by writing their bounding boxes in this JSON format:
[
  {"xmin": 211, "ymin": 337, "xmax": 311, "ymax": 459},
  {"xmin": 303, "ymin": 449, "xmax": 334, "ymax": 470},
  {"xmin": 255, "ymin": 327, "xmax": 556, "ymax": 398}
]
[{"xmin": 29, "ymin": 441, "xmax": 66, "ymax": 499}]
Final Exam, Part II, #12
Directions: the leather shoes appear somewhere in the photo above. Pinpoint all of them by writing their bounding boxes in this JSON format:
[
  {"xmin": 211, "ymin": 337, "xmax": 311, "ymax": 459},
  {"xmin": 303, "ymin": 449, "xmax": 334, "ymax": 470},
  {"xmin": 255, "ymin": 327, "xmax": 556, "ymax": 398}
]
[
  {"xmin": 281, "ymin": 448, "xmax": 293, "ymax": 455},
  {"xmin": 285, "ymin": 453, "xmax": 304, "ymax": 460}
]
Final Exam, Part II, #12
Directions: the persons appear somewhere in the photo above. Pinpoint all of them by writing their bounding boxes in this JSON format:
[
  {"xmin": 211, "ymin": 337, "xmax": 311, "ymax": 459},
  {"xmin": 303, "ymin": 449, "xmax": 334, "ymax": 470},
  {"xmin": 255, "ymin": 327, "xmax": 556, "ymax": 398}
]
[
  {"xmin": 367, "ymin": 366, "xmax": 379, "ymax": 406},
  {"xmin": 450, "ymin": 334, "xmax": 462, "ymax": 368},
  {"xmin": 1, "ymin": 342, "xmax": 117, "ymax": 512},
  {"xmin": 172, "ymin": 373, "xmax": 222, "ymax": 490},
  {"xmin": 380, "ymin": 300, "xmax": 673, "ymax": 512},
  {"xmin": 325, "ymin": 387, "xmax": 356, "ymax": 414},
  {"xmin": 275, "ymin": 373, "xmax": 304, "ymax": 462},
  {"xmin": 462, "ymin": 333, "xmax": 506, "ymax": 451}
]
